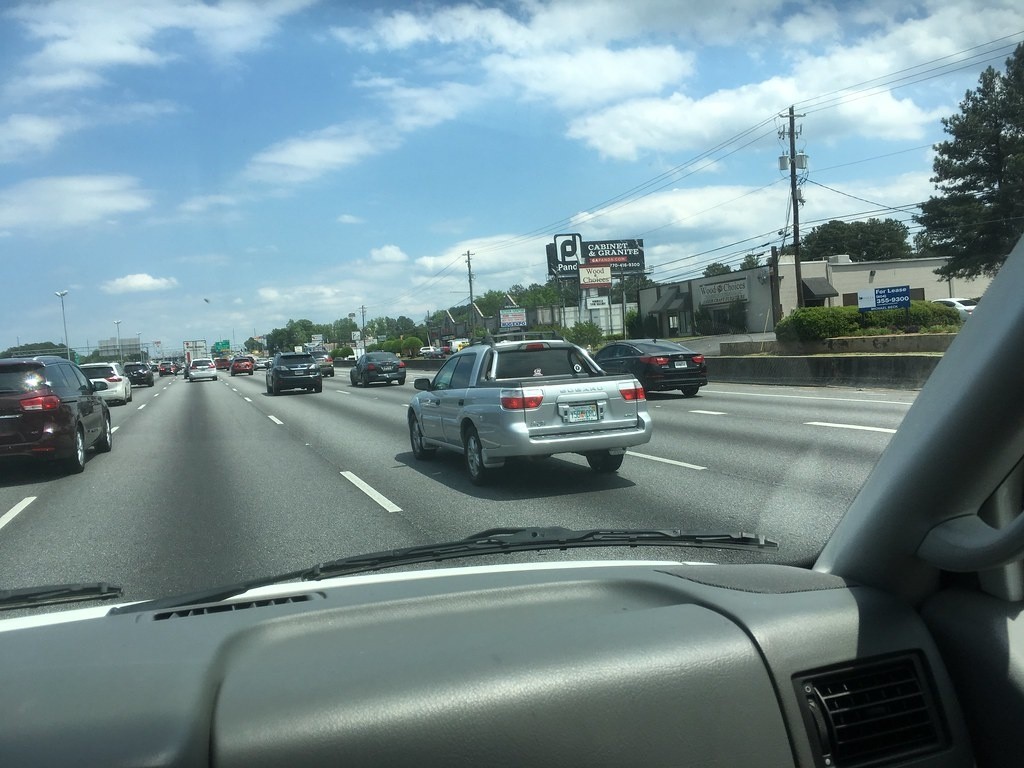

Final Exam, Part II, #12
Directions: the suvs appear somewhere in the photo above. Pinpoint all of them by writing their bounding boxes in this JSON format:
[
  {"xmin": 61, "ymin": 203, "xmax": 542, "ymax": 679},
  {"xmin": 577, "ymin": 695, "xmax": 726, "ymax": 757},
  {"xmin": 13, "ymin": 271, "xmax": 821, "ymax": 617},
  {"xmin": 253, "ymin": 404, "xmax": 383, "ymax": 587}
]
[{"xmin": 264, "ymin": 351, "xmax": 323, "ymax": 396}]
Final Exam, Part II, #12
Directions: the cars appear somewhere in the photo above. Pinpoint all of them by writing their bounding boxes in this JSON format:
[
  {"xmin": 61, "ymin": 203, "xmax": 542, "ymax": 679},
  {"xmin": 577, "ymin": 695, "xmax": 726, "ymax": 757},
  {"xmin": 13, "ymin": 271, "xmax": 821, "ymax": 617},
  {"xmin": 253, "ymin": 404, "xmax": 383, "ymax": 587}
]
[
  {"xmin": 148, "ymin": 353, "xmax": 268, "ymax": 380},
  {"xmin": 187, "ymin": 358, "xmax": 217, "ymax": 382},
  {"xmin": 122, "ymin": 361, "xmax": 154, "ymax": 388},
  {"xmin": 306, "ymin": 350, "xmax": 334, "ymax": 377},
  {"xmin": 929, "ymin": 297, "xmax": 979, "ymax": 316},
  {"xmin": 417, "ymin": 337, "xmax": 470, "ymax": 359},
  {"xmin": 229, "ymin": 357, "xmax": 253, "ymax": 376},
  {"xmin": 349, "ymin": 351, "xmax": 406, "ymax": 388},
  {"xmin": 77, "ymin": 362, "xmax": 133, "ymax": 406},
  {"xmin": 592, "ymin": 338, "xmax": 708, "ymax": 398},
  {"xmin": 0, "ymin": 352, "xmax": 113, "ymax": 477}
]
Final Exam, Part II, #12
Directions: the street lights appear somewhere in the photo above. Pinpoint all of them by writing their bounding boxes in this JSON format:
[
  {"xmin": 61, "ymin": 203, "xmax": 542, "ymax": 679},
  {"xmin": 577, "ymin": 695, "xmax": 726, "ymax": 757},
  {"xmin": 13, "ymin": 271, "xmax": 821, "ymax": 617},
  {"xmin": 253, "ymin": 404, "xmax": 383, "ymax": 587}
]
[
  {"xmin": 54, "ymin": 289, "xmax": 71, "ymax": 360},
  {"xmin": 136, "ymin": 332, "xmax": 142, "ymax": 362},
  {"xmin": 114, "ymin": 320, "xmax": 123, "ymax": 368}
]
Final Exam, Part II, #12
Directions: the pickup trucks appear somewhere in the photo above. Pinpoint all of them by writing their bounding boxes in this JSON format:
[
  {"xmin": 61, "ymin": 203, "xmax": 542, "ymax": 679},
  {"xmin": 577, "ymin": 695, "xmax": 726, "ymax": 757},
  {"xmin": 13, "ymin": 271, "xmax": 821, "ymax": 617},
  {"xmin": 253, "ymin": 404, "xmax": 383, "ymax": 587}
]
[{"xmin": 407, "ymin": 327, "xmax": 654, "ymax": 487}]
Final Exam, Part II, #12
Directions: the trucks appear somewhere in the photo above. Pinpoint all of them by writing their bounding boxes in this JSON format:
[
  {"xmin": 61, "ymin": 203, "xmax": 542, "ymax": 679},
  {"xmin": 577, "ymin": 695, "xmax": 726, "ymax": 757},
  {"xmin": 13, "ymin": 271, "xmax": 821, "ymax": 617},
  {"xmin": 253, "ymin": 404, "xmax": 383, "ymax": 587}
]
[{"xmin": 182, "ymin": 339, "xmax": 208, "ymax": 367}]
